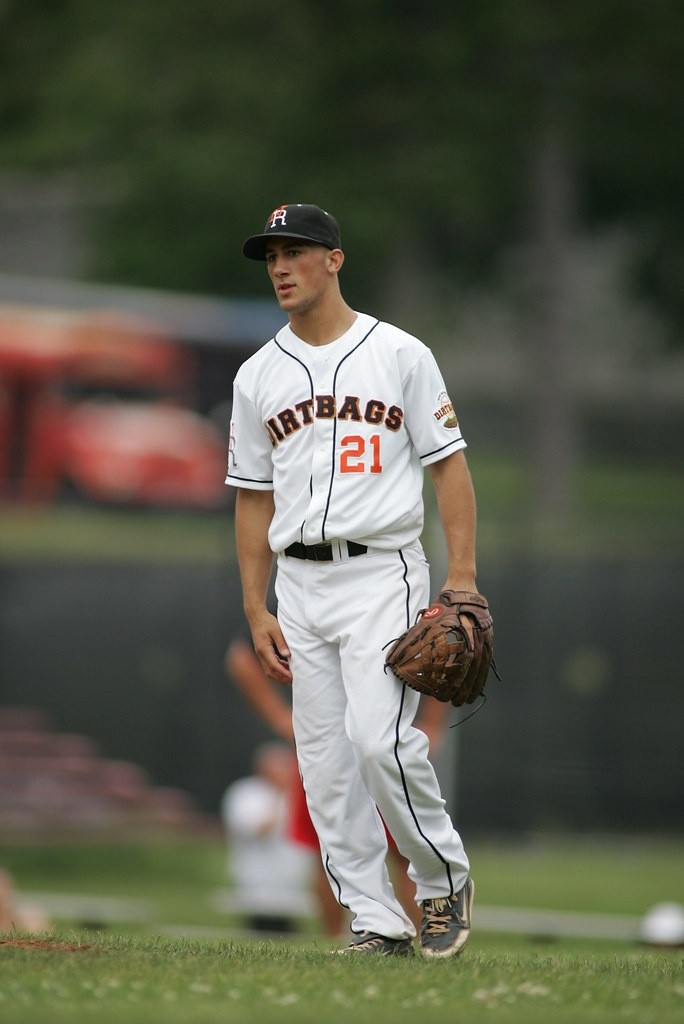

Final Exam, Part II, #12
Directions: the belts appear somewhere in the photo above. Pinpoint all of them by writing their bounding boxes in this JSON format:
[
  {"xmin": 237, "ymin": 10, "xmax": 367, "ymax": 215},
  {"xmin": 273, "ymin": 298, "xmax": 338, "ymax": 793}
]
[{"xmin": 285, "ymin": 542, "xmax": 367, "ymax": 561}]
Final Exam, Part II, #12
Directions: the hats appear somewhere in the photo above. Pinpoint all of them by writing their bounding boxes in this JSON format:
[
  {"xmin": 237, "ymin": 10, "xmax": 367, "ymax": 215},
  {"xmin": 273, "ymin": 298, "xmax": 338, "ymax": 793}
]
[{"xmin": 243, "ymin": 204, "xmax": 340, "ymax": 261}]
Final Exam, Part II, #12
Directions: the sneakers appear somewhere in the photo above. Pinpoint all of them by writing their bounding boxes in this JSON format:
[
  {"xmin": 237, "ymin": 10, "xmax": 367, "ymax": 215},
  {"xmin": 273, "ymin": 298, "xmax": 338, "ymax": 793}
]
[
  {"xmin": 324, "ymin": 929, "xmax": 416, "ymax": 962},
  {"xmin": 419, "ymin": 875, "xmax": 475, "ymax": 962}
]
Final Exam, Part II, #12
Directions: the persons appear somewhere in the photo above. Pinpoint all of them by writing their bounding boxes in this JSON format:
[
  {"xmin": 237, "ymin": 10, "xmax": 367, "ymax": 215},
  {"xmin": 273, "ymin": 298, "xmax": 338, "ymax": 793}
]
[
  {"xmin": 225, "ymin": 603, "xmax": 446, "ymax": 940},
  {"xmin": 218, "ymin": 740, "xmax": 317, "ymax": 934},
  {"xmin": 226, "ymin": 202, "xmax": 494, "ymax": 959}
]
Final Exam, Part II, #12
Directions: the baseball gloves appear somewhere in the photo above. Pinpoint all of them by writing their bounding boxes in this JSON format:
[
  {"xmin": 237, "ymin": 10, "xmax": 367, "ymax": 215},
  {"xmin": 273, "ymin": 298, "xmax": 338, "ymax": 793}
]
[{"xmin": 386, "ymin": 590, "xmax": 498, "ymax": 707}]
